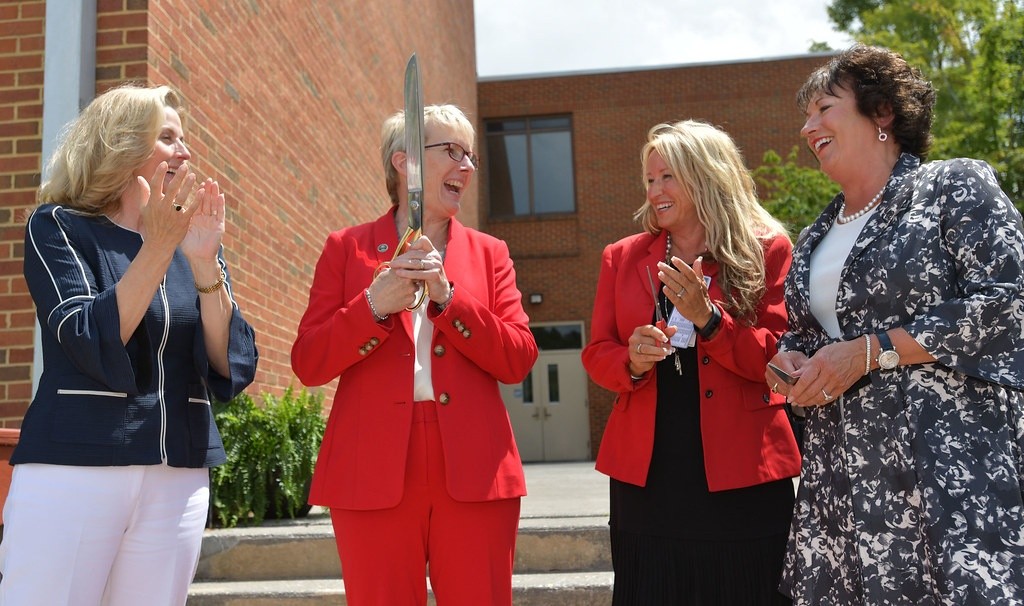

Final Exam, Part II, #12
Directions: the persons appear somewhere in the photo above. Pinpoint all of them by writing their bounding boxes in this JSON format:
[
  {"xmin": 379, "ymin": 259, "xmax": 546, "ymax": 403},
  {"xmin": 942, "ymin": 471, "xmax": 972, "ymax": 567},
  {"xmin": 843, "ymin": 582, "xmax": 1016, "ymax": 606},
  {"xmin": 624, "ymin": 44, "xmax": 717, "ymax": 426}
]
[
  {"xmin": 765, "ymin": 44, "xmax": 1024, "ymax": 606},
  {"xmin": 289, "ymin": 104, "xmax": 538, "ymax": 606},
  {"xmin": 582, "ymin": 121, "xmax": 793, "ymax": 606},
  {"xmin": 0, "ymin": 83, "xmax": 260, "ymax": 606}
]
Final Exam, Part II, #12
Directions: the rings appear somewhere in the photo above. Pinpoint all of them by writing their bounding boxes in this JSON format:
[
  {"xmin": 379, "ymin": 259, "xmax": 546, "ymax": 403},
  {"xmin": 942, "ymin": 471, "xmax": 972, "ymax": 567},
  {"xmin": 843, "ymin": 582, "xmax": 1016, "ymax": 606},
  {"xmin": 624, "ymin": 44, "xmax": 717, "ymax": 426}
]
[
  {"xmin": 637, "ymin": 344, "xmax": 643, "ymax": 356},
  {"xmin": 677, "ymin": 288, "xmax": 683, "ymax": 298},
  {"xmin": 171, "ymin": 203, "xmax": 183, "ymax": 212},
  {"xmin": 821, "ymin": 389, "xmax": 834, "ymax": 401},
  {"xmin": 772, "ymin": 383, "xmax": 778, "ymax": 394}
]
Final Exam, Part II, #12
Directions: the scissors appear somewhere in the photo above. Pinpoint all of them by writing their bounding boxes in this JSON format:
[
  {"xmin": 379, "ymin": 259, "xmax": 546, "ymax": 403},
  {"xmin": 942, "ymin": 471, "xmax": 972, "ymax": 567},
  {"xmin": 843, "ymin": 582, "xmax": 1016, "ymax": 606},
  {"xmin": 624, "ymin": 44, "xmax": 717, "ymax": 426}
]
[
  {"xmin": 768, "ymin": 363, "xmax": 801, "ymax": 399},
  {"xmin": 369, "ymin": 52, "xmax": 430, "ymax": 312},
  {"xmin": 646, "ymin": 264, "xmax": 672, "ymax": 350}
]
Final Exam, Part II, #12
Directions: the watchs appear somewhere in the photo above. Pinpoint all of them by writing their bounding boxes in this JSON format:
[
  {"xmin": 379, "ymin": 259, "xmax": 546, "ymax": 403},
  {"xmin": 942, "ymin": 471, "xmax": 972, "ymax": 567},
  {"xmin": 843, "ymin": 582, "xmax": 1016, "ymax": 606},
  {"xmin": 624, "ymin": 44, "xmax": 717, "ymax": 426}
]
[{"xmin": 874, "ymin": 330, "xmax": 900, "ymax": 372}]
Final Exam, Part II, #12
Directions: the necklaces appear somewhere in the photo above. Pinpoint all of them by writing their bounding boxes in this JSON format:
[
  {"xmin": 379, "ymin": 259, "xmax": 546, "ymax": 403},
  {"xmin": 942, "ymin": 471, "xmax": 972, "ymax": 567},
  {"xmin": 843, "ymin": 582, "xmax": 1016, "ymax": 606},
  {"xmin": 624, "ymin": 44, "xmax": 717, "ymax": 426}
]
[
  {"xmin": 838, "ymin": 185, "xmax": 885, "ymax": 224},
  {"xmin": 659, "ymin": 231, "xmax": 709, "ymax": 376}
]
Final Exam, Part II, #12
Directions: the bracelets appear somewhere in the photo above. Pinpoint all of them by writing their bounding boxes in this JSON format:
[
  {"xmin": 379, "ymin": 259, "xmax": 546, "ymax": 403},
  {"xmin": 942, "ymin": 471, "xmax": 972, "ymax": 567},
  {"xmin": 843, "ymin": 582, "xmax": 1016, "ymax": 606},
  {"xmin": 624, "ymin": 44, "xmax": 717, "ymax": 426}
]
[
  {"xmin": 435, "ymin": 286, "xmax": 454, "ymax": 311},
  {"xmin": 861, "ymin": 333, "xmax": 871, "ymax": 375},
  {"xmin": 366, "ymin": 289, "xmax": 389, "ymax": 323},
  {"xmin": 693, "ymin": 303, "xmax": 721, "ymax": 338},
  {"xmin": 193, "ymin": 265, "xmax": 227, "ymax": 294},
  {"xmin": 630, "ymin": 374, "xmax": 643, "ymax": 382}
]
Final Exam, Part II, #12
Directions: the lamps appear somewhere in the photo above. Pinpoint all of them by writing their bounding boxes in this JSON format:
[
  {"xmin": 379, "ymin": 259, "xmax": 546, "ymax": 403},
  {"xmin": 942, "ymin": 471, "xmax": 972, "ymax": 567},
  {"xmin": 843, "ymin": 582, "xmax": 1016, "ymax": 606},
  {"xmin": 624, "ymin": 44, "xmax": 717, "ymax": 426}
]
[{"xmin": 530, "ymin": 293, "xmax": 544, "ymax": 304}]
